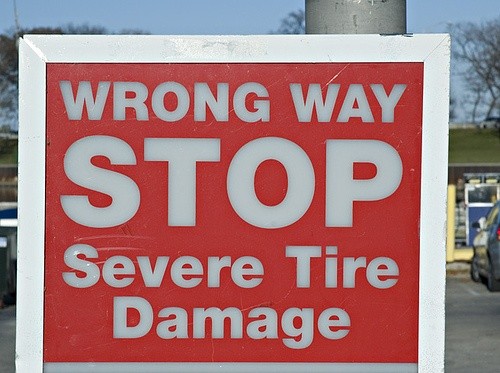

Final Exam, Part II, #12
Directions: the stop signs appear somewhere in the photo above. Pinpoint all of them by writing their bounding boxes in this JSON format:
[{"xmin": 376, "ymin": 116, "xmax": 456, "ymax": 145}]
[{"xmin": 15, "ymin": 35, "xmax": 450, "ymax": 373}]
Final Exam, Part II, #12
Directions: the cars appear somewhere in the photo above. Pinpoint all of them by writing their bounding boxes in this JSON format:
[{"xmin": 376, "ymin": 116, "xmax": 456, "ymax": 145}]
[{"xmin": 471, "ymin": 200, "xmax": 500, "ymax": 291}]
